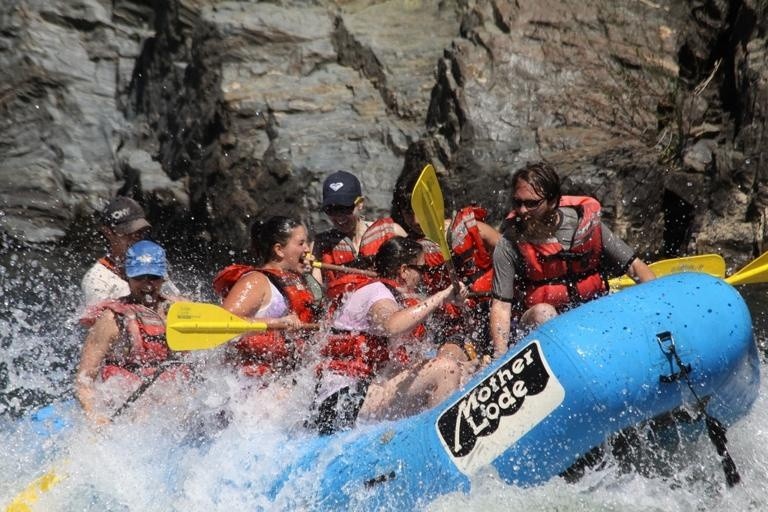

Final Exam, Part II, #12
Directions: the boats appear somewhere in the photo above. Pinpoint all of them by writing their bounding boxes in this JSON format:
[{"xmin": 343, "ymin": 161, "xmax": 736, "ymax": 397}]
[{"xmin": 28, "ymin": 272, "xmax": 760, "ymax": 512}]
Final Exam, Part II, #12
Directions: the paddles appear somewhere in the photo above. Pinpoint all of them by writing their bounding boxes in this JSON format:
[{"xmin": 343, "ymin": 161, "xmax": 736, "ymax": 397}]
[
  {"xmin": 166, "ymin": 301, "xmax": 388, "ymax": 352},
  {"xmin": 5, "ymin": 351, "xmax": 180, "ymax": 512},
  {"xmin": 410, "ymin": 164, "xmax": 483, "ymax": 362},
  {"xmin": 468, "ymin": 253, "xmax": 727, "ymax": 300},
  {"xmin": 724, "ymin": 250, "xmax": 768, "ymax": 286}
]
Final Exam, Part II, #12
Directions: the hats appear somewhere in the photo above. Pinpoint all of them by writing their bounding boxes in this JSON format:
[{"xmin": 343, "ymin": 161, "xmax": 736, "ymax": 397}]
[
  {"xmin": 124, "ymin": 240, "xmax": 168, "ymax": 278},
  {"xmin": 320, "ymin": 168, "xmax": 361, "ymax": 208},
  {"xmin": 104, "ymin": 196, "xmax": 153, "ymax": 237}
]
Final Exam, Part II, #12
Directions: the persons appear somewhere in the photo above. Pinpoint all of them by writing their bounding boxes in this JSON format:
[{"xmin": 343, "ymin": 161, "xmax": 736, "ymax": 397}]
[
  {"xmin": 218, "ymin": 214, "xmax": 332, "ymax": 387},
  {"xmin": 304, "ymin": 239, "xmax": 460, "ymax": 431},
  {"xmin": 490, "ymin": 163, "xmax": 657, "ymax": 361},
  {"xmin": 397, "ymin": 175, "xmax": 505, "ymax": 363},
  {"xmin": 78, "ymin": 194, "xmax": 179, "ymax": 332},
  {"xmin": 71, "ymin": 239, "xmax": 200, "ymax": 429},
  {"xmin": 312, "ymin": 173, "xmax": 410, "ymax": 309}
]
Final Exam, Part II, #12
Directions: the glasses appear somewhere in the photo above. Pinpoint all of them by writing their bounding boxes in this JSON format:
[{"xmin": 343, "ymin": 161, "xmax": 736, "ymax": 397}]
[{"xmin": 512, "ymin": 196, "xmax": 546, "ymax": 208}]
